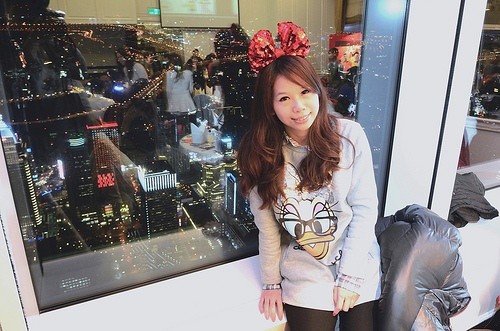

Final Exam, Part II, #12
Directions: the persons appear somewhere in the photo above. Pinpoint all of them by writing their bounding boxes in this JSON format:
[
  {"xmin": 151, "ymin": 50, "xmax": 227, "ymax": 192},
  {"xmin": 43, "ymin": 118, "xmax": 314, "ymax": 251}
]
[
  {"xmin": 236, "ymin": 54, "xmax": 383, "ymax": 331},
  {"xmin": 0, "ymin": 0, "xmax": 362, "ymax": 187}
]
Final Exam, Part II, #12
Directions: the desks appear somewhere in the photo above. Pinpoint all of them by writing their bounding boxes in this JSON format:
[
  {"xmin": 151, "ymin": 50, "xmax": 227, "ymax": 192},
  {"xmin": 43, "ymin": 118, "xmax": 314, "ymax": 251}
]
[{"xmin": 178, "ymin": 128, "xmax": 226, "ymax": 200}]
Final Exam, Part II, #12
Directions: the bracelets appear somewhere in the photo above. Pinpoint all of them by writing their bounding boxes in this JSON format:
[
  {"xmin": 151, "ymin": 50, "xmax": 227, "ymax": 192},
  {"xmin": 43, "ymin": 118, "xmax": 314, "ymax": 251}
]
[{"xmin": 261, "ymin": 283, "xmax": 281, "ymax": 290}]
